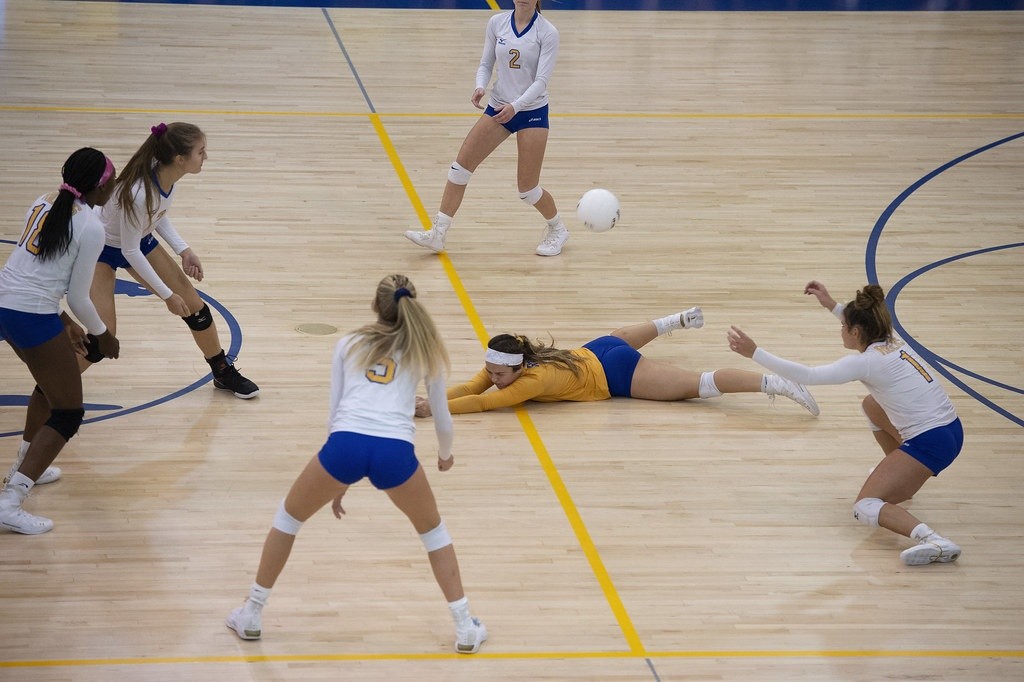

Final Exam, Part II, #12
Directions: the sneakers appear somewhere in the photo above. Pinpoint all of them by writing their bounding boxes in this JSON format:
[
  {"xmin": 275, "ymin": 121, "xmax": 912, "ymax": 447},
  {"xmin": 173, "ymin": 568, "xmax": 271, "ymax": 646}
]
[
  {"xmin": 536, "ymin": 218, "xmax": 569, "ymax": 257},
  {"xmin": 212, "ymin": 364, "xmax": 259, "ymax": 399},
  {"xmin": 0, "ymin": 484, "xmax": 53, "ymax": 535},
  {"xmin": 455, "ymin": 618, "xmax": 487, "ymax": 654},
  {"xmin": 900, "ymin": 530, "xmax": 962, "ymax": 565},
  {"xmin": 225, "ymin": 598, "xmax": 264, "ymax": 639},
  {"xmin": 667, "ymin": 306, "xmax": 705, "ymax": 337},
  {"xmin": 3, "ymin": 449, "xmax": 61, "ymax": 485},
  {"xmin": 405, "ymin": 219, "xmax": 448, "ymax": 253},
  {"xmin": 767, "ymin": 374, "xmax": 819, "ymax": 416}
]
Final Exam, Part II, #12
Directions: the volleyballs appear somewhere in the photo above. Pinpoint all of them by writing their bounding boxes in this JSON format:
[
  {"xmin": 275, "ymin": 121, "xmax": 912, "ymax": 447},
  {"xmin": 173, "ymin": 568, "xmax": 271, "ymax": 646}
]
[{"xmin": 574, "ymin": 188, "xmax": 622, "ymax": 234}]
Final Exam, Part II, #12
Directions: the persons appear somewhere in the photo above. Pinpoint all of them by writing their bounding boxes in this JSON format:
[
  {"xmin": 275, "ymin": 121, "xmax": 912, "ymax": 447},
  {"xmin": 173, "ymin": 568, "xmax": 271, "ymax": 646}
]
[
  {"xmin": 405, "ymin": 0, "xmax": 571, "ymax": 256},
  {"xmin": 0, "ymin": 147, "xmax": 120, "ymax": 534},
  {"xmin": 75, "ymin": 121, "xmax": 259, "ymax": 399},
  {"xmin": 726, "ymin": 279, "xmax": 964, "ymax": 565},
  {"xmin": 415, "ymin": 306, "xmax": 821, "ymax": 418},
  {"xmin": 226, "ymin": 274, "xmax": 486, "ymax": 654}
]
